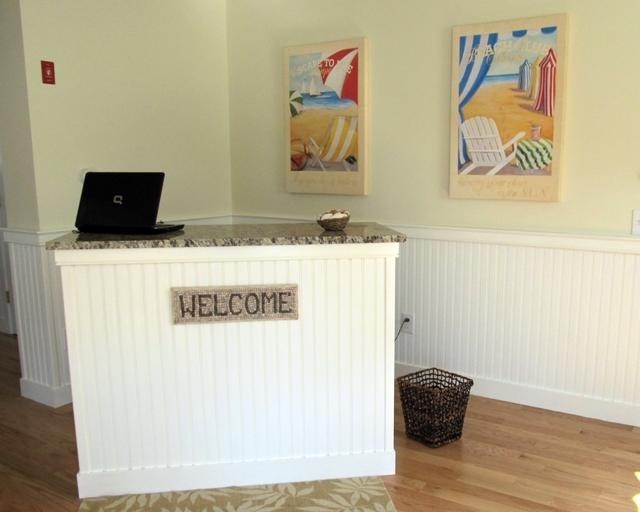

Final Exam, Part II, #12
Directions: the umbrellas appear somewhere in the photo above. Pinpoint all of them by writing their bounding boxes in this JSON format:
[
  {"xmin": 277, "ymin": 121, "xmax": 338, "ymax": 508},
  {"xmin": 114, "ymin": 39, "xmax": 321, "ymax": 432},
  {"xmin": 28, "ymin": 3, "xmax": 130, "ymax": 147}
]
[
  {"xmin": 318, "ymin": 47, "xmax": 357, "ymax": 105},
  {"xmin": 289, "ymin": 90, "xmax": 304, "ymax": 118}
]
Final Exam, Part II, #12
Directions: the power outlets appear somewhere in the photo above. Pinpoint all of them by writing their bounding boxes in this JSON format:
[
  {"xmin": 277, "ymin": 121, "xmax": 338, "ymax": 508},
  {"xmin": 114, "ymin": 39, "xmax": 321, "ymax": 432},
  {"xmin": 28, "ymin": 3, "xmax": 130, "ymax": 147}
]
[{"xmin": 398, "ymin": 312, "xmax": 415, "ymax": 335}]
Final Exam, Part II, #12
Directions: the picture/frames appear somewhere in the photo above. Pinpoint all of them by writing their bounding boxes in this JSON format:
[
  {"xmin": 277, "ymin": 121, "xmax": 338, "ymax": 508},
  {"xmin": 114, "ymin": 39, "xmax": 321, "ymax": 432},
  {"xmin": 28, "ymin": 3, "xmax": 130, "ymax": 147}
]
[
  {"xmin": 448, "ymin": 13, "xmax": 569, "ymax": 204},
  {"xmin": 282, "ymin": 39, "xmax": 368, "ymax": 196}
]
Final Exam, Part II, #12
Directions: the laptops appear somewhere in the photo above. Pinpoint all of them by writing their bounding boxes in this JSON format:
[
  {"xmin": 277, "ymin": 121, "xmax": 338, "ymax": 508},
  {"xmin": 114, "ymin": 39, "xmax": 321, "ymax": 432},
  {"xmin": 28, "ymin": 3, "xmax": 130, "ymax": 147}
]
[{"xmin": 73, "ymin": 170, "xmax": 184, "ymax": 240}]
